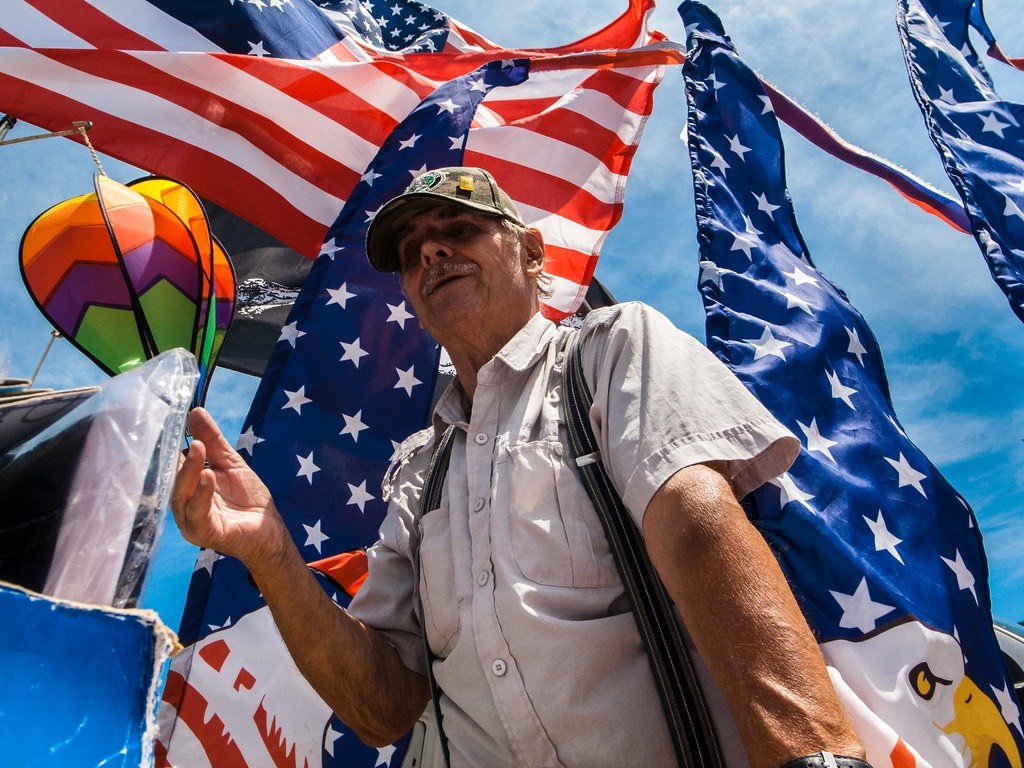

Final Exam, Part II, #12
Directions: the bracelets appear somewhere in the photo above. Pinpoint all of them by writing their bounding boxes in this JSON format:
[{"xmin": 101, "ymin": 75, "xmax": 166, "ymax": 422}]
[{"xmin": 782, "ymin": 751, "xmax": 873, "ymax": 768}]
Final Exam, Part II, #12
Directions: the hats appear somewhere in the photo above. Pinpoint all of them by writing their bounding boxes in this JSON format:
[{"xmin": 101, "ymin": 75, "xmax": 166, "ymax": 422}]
[{"xmin": 366, "ymin": 166, "xmax": 525, "ymax": 272}]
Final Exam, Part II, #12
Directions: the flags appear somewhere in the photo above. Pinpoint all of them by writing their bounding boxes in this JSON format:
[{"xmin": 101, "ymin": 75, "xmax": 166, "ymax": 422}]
[{"xmin": 0, "ymin": 0, "xmax": 1024, "ymax": 768}]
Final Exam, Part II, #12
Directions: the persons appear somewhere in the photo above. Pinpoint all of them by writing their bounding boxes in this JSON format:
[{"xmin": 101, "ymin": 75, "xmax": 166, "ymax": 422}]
[{"xmin": 168, "ymin": 167, "xmax": 874, "ymax": 768}]
